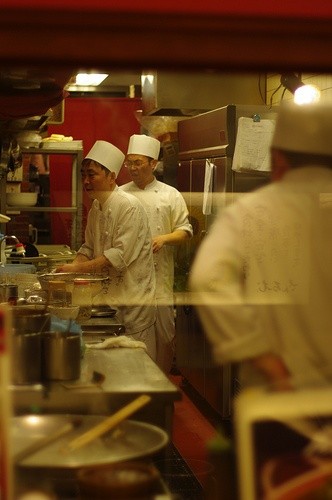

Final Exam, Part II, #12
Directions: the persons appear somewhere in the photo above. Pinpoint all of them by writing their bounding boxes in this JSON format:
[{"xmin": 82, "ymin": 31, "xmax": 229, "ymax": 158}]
[
  {"xmin": 55, "ymin": 140, "xmax": 156, "ymax": 364},
  {"xmin": 118, "ymin": 134, "xmax": 193, "ymax": 374},
  {"xmin": 187, "ymin": 97, "xmax": 332, "ymax": 392}
]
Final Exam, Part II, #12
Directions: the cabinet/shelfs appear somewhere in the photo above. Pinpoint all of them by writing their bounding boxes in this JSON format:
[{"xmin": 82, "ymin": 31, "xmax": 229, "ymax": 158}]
[
  {"xmin": 6, "ymin": 96, "xmax": 142, "ymax": 243},
  {"xmin": 0, "ymin": 147, "xmax": 84, "ymax": 250}
]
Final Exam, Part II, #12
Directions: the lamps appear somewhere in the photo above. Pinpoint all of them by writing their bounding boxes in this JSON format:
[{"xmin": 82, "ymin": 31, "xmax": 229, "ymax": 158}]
[{"xmin": 279, "ymin": 71, "xmax": 322, "ymax": 106}]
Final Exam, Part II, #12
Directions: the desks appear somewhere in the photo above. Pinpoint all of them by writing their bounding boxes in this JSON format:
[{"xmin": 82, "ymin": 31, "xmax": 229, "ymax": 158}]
[{"xmin": 10, "ymin": 245, "xmax": 182, "ymax": 446}]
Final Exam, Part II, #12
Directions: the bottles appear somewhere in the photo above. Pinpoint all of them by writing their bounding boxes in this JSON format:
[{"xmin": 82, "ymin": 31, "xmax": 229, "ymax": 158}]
[{"xmin": 72, "ymin": 280, "xmax": 93, "ymax": 320}]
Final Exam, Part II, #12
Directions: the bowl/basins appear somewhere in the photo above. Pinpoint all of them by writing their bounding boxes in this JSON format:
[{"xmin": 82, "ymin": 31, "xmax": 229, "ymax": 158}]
[
  {"xmin": 6, "ymin": 192, "xmax": 38, "ymax": 206},
  {"xmin": 49, "ymin": 303, "xmax": 81, "ymax": 320}
]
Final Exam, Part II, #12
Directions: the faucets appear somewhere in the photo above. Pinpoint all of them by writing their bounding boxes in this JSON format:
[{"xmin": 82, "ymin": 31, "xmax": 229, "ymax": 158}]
[{"xmin": 0, "ymin": 235, "xmax": 26, "ymax": 262}]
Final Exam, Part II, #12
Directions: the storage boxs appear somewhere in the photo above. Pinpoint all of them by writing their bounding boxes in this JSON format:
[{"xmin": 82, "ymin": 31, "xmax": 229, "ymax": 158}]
[
  {"xmin": 7, "ymin": 162, "xmax": 23, "ymax": 182},
  {"xmin": 6, "ymin": 182, "xmax": 21, "ymax": 215},
  {"xmin": 41, "ymin": 139, "xmax": 83, "ymax": 151}
]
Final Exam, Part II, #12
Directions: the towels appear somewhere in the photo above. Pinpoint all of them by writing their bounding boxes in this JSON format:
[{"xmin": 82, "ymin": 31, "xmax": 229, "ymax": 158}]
[{"xmin": 201, "ymin": 158, "xmax": 217, "ymax": 215}]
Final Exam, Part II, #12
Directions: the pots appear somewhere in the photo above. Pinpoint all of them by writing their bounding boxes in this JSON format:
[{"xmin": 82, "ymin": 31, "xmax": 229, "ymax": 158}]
[
  {"xmin": 0, "ymin": 285, "xmax": 18, "ymax": 303},
  {"xmin": 37, "ymin": 272, "xmax": 110, "ymax": 298},
  {"xmin": 15, "ymin": 308, "xmax": 51, "ymax": 334},
  {"xmin": 22, "ymin": 331, "xmax": 82, "ymax": 382},
  {"xmin": 13, "ymin": 413, "xmax": 168, "ymax": 500}
]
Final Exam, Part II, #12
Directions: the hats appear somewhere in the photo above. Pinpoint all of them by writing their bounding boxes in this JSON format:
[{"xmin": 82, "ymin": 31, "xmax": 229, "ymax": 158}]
[
  {"xmin": 269, "ymin": 100, "xmax": 332, "ymax": 155},
  {"xmin": 83, "ymin": 139, "xmax": 126, "ymax": 180},
  {"xmin": 128, "ymin": 134, "xmax": 160, "ymax": 160}
]
